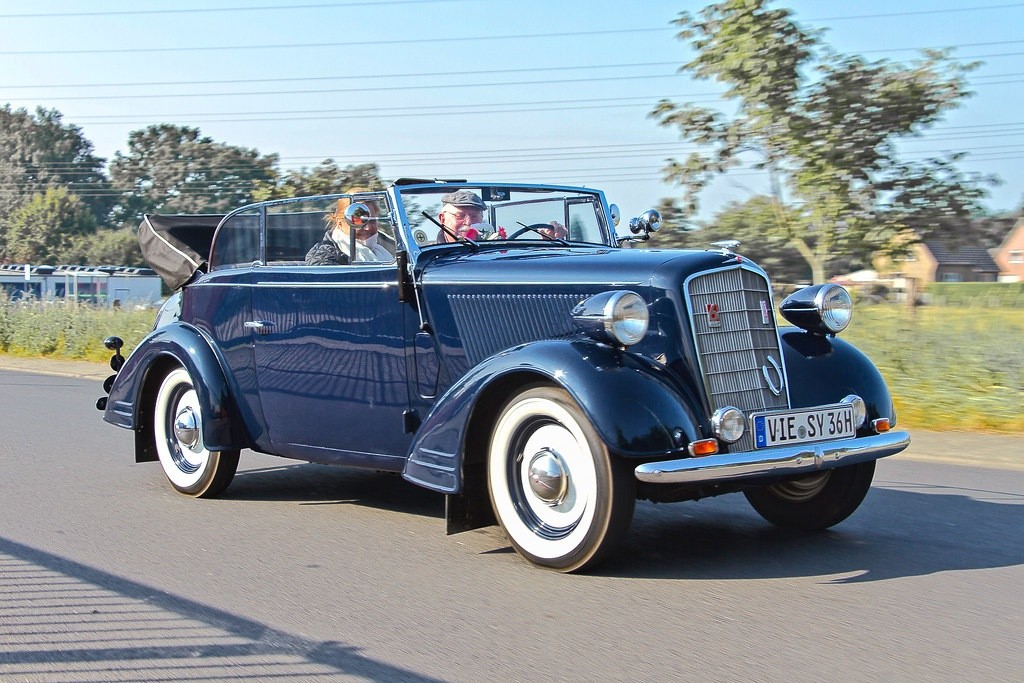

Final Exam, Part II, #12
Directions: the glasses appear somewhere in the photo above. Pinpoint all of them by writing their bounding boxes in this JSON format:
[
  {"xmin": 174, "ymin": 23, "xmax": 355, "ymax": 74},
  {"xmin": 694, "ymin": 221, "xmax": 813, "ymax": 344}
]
[{"xmin": 444, "ymin": 209, "xmax": 483, "ymax": 222}]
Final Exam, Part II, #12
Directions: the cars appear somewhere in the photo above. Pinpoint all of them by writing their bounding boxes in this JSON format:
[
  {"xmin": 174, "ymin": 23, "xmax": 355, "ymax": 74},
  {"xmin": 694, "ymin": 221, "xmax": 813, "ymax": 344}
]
[{"xmin": 94, "ymin": 177, "xmax": 911, "ymax": 574}]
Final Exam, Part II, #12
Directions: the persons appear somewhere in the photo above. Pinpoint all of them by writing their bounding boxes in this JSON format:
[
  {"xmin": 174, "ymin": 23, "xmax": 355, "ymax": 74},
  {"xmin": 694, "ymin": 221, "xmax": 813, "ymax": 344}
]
[
  {"xmin": 420, "ymin": 190, "xmax": 568, "ymax": 247},
  {"xmin": 303, "ymin": 186, "xmax": 395, "ymax": 265}
]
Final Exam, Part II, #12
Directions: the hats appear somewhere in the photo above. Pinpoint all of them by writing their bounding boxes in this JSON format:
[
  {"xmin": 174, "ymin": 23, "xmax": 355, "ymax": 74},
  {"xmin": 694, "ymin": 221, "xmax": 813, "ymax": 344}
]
[{"xmin": 444, "ymin": 190, "xmax": 488, "ymax": 211}]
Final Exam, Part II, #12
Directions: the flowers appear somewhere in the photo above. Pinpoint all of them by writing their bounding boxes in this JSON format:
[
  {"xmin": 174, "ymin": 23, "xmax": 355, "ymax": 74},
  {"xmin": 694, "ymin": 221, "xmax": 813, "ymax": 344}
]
[
  {"xmin": 465, "ymin": 228, "xmax": 479, "ymax": 241},
  {"xmin": 491, "ymin": 226, "xmax": 507, "ymax": 241}
]
